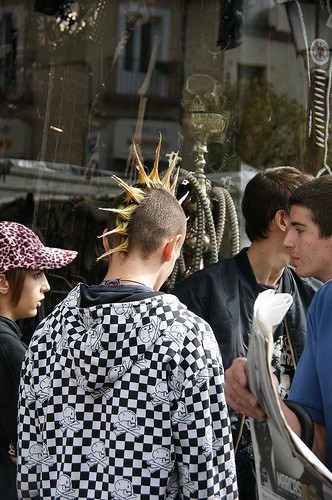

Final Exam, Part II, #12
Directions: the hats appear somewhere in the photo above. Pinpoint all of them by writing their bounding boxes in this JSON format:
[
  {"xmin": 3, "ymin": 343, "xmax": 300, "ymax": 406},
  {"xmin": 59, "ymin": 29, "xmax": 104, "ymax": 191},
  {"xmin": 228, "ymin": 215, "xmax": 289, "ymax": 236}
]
[{"xmin": 0, "ymin": 221, "xmax": 78, "ymax": 274}]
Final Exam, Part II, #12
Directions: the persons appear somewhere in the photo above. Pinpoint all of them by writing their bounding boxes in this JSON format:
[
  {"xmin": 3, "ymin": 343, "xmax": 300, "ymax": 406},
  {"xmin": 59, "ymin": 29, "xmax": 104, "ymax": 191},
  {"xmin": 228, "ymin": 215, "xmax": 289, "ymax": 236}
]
[
  {"xmin": 0, "ymin": 220, "xmax": 78, "ymax": 500},
  {"xmin": 16, "ymin": 133, "xmax": 239, "ymax": 500},
  {"xmin": 167, "ymin": 167, "xmax": 332, "ymax": 500}
]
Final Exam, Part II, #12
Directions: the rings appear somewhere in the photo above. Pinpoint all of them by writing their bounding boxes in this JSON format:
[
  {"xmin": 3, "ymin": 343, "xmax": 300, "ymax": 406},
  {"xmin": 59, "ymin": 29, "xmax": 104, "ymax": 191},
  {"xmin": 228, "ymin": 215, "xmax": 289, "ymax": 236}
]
[{"xmin": 231, "ymin": 391, "xmax": 236, "ymax": 398}]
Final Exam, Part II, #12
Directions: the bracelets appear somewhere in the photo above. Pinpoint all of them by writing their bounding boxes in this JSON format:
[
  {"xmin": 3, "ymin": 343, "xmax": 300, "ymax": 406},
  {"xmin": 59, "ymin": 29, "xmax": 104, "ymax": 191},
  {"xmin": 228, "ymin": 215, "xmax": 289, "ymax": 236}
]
[{"xmin": 281, "ymin": 400, "xmax": 315, "ymax": 450}]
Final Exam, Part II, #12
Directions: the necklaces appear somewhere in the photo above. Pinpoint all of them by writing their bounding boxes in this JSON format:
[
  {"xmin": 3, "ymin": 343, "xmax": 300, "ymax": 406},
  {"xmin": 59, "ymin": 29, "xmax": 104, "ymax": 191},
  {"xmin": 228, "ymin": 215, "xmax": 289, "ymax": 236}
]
[{"xmin": 102, "ymin": 277, "xmax": 147, "ymax": 287}]
